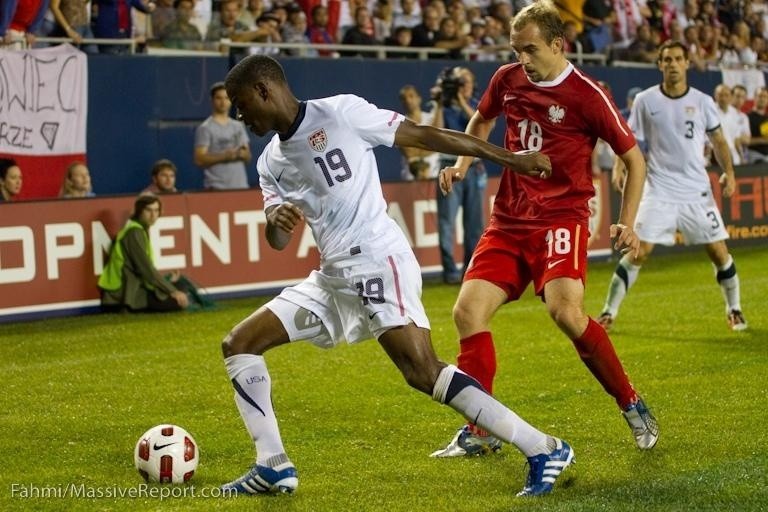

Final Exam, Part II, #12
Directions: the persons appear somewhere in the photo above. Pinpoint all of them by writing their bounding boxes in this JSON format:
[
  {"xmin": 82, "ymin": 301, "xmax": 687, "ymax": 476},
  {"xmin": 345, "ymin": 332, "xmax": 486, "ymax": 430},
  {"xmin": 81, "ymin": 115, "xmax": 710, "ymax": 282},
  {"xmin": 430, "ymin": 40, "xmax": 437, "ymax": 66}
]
[
  {"xmin": 217, "ymin": 53, "xmax": 580, "ymax": 500},
  {"xmin": 0, "ymin": 2, "xmax": 768, "ymax": 315},
  {"xmin": 594, "ymin": 40, "xmax": 752, "ymax": 334},
  {"xmin": 428, "ymin": 2, "xmax": 661, "ymax": 459}
]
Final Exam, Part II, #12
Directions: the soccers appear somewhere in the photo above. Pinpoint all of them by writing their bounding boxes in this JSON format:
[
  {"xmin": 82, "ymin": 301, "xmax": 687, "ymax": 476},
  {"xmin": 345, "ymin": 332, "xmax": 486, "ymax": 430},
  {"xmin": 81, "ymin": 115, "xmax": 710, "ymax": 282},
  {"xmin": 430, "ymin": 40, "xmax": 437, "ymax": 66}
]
[{"xmin": 134, "ymin": 425, "xmax": 198, "ymax": 484}]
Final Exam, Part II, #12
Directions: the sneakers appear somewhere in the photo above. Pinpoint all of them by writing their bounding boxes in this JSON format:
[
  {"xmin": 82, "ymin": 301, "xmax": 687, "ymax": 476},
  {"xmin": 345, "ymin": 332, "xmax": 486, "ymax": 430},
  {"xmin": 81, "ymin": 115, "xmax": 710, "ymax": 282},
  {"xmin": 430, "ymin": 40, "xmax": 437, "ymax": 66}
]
[
  {"xmin": 725, "ymin": 311, "xmax": 747, "ymax": 332},
  {"xmin": 622, "ymin": 394, "xmax": 659, "ymax": 450},
  {"xmin": 216, "ymin": 464, "xmax": 298, "ymax": 495},
  {"xmin": 515, "ymin": 437, "xmax": 575, "ymax": 496},
  {"xmin": 428, "ymin": 427, "xmax": 503, "ymax": 458},
  {"xmin": 599, "ymin": 313, "xmax": 611, "ymax": 332}
]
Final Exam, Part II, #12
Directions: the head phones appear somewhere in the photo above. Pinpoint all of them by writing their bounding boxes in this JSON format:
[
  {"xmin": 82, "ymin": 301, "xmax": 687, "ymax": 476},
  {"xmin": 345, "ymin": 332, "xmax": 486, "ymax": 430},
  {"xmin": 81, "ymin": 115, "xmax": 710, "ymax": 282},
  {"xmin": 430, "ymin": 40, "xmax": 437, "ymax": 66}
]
[{"xmin": 473, "ymin": 80, "xmax": 482, "ymax": 98}]
